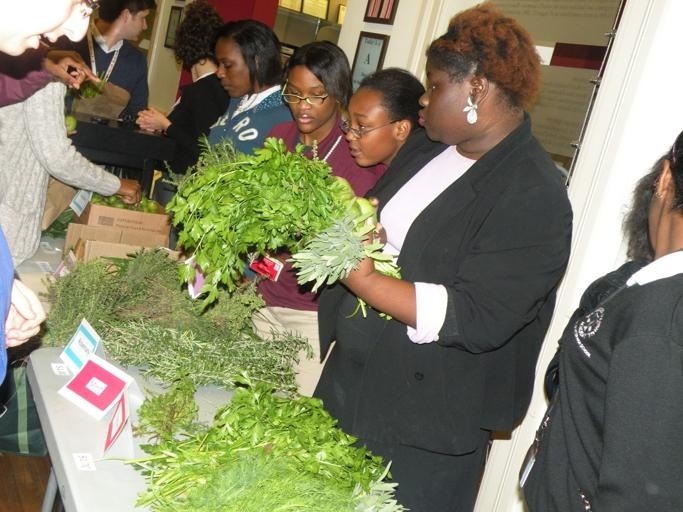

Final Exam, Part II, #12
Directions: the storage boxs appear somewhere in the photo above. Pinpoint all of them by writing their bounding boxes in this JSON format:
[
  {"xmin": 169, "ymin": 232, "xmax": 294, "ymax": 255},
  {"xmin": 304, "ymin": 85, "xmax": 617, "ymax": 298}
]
[{"xmin": 64, "ymin": 205, "xmax": 180, "ymax": 268}]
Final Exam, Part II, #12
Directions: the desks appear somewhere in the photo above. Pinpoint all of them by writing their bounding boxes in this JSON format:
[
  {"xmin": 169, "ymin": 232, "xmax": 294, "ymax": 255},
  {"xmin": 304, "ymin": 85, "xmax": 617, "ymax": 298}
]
[{"xmin": 28, "ymin": 339, "xmax": 405, "ymax": 512}]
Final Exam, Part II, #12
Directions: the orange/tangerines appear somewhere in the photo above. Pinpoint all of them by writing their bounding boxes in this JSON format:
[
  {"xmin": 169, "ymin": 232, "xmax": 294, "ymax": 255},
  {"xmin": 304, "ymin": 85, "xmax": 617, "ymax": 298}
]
[
  {"xmin": 89, "ymin": 192, "xmax": 157, "ymax": 214},
  {"xmin": 65, "ymin": 116, "xmax": 77, "ymax": 132}
]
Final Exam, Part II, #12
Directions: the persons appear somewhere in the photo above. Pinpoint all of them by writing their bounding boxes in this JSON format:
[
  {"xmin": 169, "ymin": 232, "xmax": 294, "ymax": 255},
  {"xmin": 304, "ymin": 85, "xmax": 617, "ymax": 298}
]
[
  {"xmin": 310, "ymin": 7, "xmax": 574, "ymax": 512},
  {"xmin": 338, "ymin": 67, "xmax": 426, "ymax": 168},
  {"xmin": 518, "ymin": 132, "xmax": 682, "ymax": 512},
  {"xmin": 135, "ymin": 2, "xmax": 226, "ymax": 251},
  {"xmin": 542, "ymin": 150, "xmax": 669, "ymax": 407},
  {"xmin": 237, "ymin": 39, "xmax": 387, "ymax": 397},
  {"xmin": 1, "ymin": 1, "xmax": 157, "ymax": 390},
  {"xmin": 168, "ymin": 16, "xmax": 297, "ymax": 296}
]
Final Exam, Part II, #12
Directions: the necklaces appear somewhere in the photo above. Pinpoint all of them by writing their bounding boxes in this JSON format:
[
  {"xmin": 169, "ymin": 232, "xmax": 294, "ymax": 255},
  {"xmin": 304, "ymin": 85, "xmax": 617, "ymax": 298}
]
[{"xmin": 320, "ymin": 134, "xmax": 342, "ymax": 162}]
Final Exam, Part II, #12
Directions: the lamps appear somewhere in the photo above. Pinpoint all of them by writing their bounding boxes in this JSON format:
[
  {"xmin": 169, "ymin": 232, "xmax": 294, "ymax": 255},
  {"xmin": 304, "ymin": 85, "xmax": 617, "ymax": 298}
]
[{"xmin": 164, "ymin": 6, "xmax": 184, "ymax": 48}]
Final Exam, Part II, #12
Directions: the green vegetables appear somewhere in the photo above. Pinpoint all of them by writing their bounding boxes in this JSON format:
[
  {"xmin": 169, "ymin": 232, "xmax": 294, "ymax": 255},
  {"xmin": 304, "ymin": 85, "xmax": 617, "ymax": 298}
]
[
  {"xmin": 163, "ymin": 133, "xmax": 401, "ymax": 320},
  {"xmin": 123, "ymin": 371, "xmax": 392, "ymax": 512}
]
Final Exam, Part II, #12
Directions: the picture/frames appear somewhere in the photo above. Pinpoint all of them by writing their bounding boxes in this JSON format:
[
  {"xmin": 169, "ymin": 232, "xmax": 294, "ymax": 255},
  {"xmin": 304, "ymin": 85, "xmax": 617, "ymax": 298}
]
[
  {"xmin": 348, "ymin": 31, "xmax": 392, "ymax": 94},
  {"xmin": 363, "ymin": 0, "xmax": 402, "ymax": 25}
]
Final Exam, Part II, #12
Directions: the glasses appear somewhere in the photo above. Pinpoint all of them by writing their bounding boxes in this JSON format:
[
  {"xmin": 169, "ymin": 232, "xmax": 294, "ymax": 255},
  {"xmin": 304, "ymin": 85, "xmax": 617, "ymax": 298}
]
[
  {"xmin": 81, "ymin": 1, "xmax": 100, "ymax": 18},
  {"xmin": 280, "ymin": 78, "xmax": 328, "ymax": 106},
  {"xmin": 339, "ymin": 118, "xmax": 403, "ymax": 140}
]
